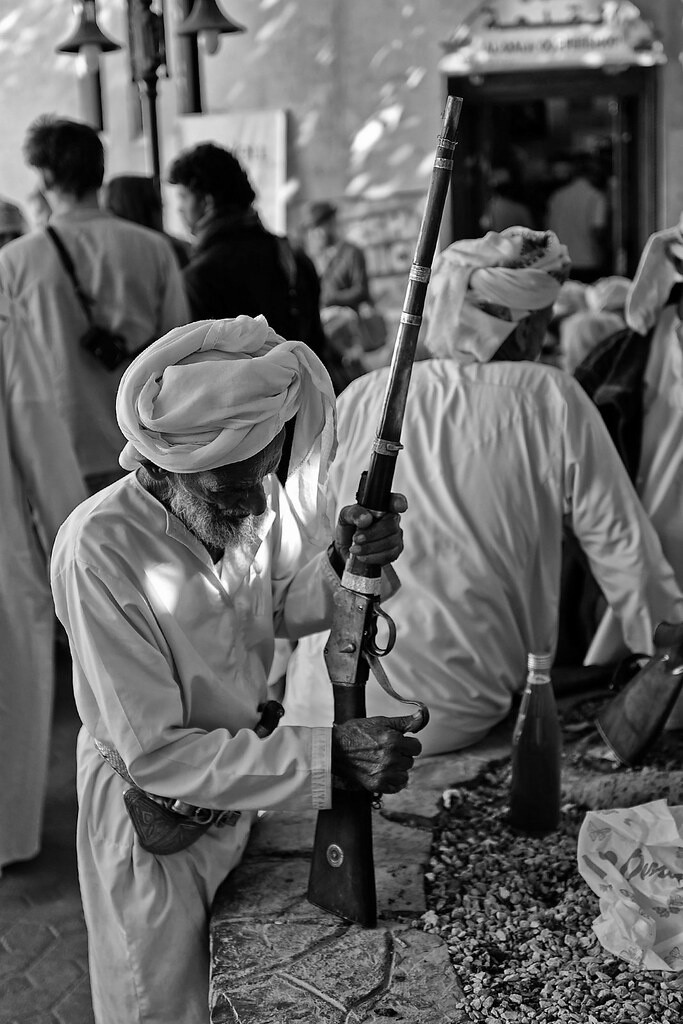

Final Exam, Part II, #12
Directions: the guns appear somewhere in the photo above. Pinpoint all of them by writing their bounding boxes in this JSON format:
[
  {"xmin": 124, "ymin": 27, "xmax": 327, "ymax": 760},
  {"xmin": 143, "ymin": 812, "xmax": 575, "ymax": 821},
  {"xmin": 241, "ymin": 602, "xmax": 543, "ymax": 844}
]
[{"xmin": 304, "ymin": 94, "xmax": 466, "ymax": 928}]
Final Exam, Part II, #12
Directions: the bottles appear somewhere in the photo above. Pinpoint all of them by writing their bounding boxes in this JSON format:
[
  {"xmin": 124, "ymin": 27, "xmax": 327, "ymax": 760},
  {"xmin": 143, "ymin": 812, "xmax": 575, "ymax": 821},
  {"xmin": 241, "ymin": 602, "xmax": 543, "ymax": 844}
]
[{"xmin": 511, "ymin": 652, "xmax": 563, "ymax": 840}]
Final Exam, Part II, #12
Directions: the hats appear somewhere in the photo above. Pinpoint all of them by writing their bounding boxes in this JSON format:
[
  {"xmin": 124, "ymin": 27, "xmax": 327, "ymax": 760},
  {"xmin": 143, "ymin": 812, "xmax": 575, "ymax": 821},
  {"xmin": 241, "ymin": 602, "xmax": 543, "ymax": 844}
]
[{"xmin": 300, "ymin": 202, "xmax": 337, "ymax": 232}]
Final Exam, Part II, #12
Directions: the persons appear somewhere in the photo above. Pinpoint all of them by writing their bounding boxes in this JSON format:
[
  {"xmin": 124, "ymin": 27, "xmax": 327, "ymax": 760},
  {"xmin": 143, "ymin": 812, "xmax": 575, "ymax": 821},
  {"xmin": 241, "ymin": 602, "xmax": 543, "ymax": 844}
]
[
  {"xmin": 0, "ymin": 116, "xmax": 683, "ymax": 866},
  {"xmin": 49, "ymin": 314, "xmax": 425, "ymax": 1024}
]
